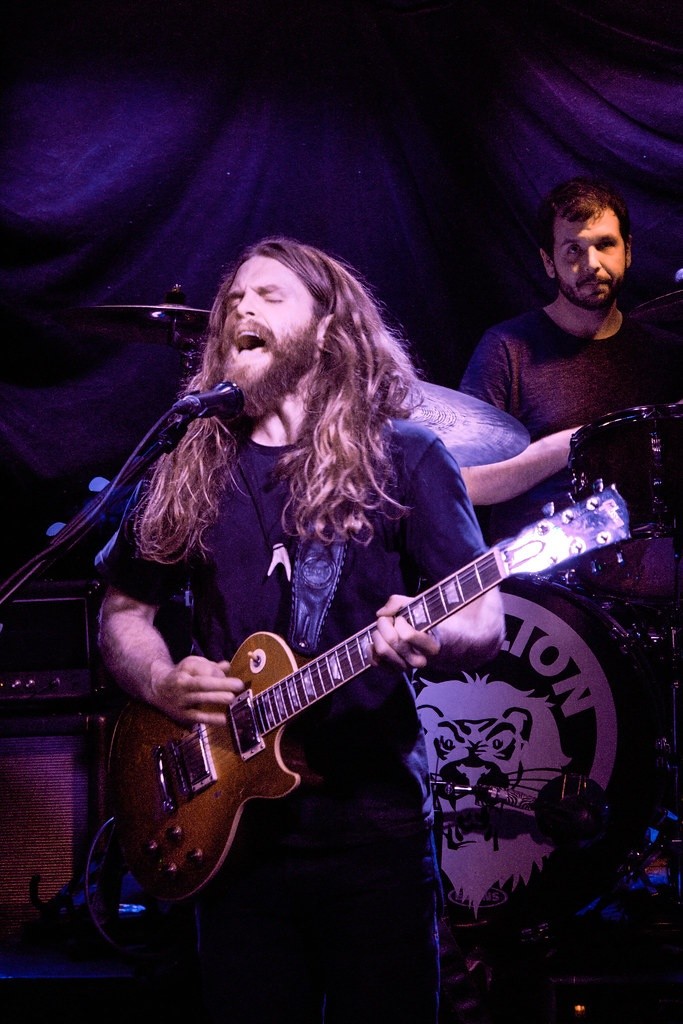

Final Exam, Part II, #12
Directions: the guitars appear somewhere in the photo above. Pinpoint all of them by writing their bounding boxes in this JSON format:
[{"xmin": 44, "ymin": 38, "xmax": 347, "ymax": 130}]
[{"xmin": 104, "ymin": 474, "xmax": 635, "ymax": 909}]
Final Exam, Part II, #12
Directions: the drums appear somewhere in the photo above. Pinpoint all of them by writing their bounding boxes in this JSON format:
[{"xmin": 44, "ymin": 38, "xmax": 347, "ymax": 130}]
[
  {"xmin": 567, "ymin": 400, "xmax": 683, "ymax": 605},
  {"xmin": 394, "ymin": 566, "xmax": 668, "ymax": 919}
]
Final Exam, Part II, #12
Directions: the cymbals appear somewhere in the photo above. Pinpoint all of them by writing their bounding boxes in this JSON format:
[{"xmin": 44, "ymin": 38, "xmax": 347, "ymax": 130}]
[
  {"xmin": 400, "ymin": 377, "xmax": 532, "ymax": 469},
  {"xmin": 65, "ymin": 299, "xmax": 212, "ymax": 332},
  {"xmin": 628, "ymin": 289, "xmax": 683, "ymax": 323}
]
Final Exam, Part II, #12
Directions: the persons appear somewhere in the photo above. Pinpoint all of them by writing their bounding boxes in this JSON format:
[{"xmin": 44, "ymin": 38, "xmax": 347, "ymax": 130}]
[
  {"xmin": 92, "ymin": 236, "xmax": 509, "ymax": 1023},
  {"xmin": 458, "ymin": 178, "xmax": 682, "ymax": 593}
]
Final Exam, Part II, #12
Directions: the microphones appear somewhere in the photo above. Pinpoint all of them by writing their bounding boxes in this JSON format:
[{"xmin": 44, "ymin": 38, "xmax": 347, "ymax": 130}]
[
  {"xmin": 553, "ymin": 795, "xmax": 609, "ymax": 845},
  {"xmin": 174, "ymin": 379, "xmax": 246, "ymax": 419}
]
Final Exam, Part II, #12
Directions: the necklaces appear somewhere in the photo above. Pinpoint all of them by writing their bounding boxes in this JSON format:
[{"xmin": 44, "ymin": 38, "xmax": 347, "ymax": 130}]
[{"xmin": 230, "ymin": 432, "xmax": 297, "ymax": 584}]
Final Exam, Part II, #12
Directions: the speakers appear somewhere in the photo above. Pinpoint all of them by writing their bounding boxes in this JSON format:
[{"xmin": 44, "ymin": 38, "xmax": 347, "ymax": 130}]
[{"xmin": 0, "ymin": 714, "xmax": 111, "ymax": 942}]
